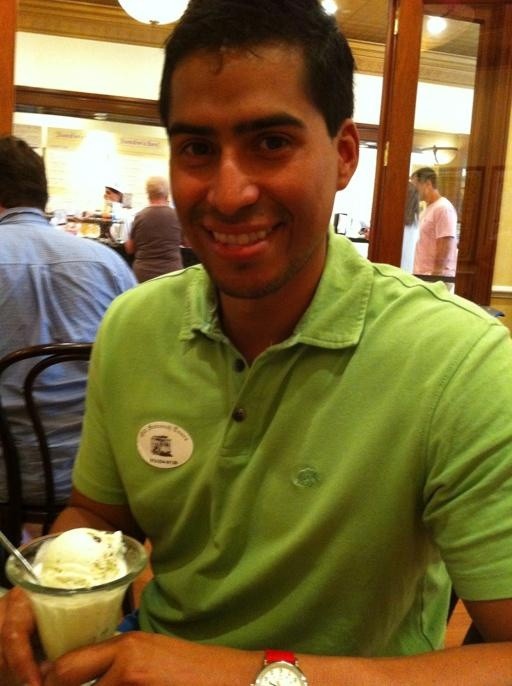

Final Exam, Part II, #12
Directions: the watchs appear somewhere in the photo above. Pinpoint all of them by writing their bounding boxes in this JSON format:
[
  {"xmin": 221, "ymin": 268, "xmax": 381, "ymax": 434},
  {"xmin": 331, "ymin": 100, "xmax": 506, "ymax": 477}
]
[{"xmin": 249, "ymin": 649, "xmax": 309, "ymax": 686}]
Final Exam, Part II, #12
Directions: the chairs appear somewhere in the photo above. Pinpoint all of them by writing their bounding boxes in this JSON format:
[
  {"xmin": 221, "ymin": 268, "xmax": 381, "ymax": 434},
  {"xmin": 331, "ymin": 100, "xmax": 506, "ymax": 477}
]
[{"xmin": 0, "ymin": 341, "xmax": 93, "ymax": 545}]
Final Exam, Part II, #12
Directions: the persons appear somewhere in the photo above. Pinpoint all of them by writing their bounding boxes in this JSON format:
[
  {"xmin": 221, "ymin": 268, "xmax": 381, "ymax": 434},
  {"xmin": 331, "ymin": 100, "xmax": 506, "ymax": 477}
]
[
  {"xmin": 125, "ymin": 175, "xmax": 183, "ymax": 284},
  {"xmin": 1, "ymin": 0, "xmax": 512, "ymax": 686},
  {"xmin": 1, "ymin": 134, "xmax": 137, "ymax": 509},
  {"xmin": 104, "ymin": 182, "xmax": 136, "ymax": 244},
  {"xmin": 400, "ymin": 167, "xmax": 457, "ymax": 292}
]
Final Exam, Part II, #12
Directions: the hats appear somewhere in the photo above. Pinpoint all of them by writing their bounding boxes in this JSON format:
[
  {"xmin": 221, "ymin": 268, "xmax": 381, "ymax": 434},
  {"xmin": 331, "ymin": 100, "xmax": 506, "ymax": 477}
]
[{"xmin": 105, "ymin": 179, "xmax": 126, "ymax": 193}]
